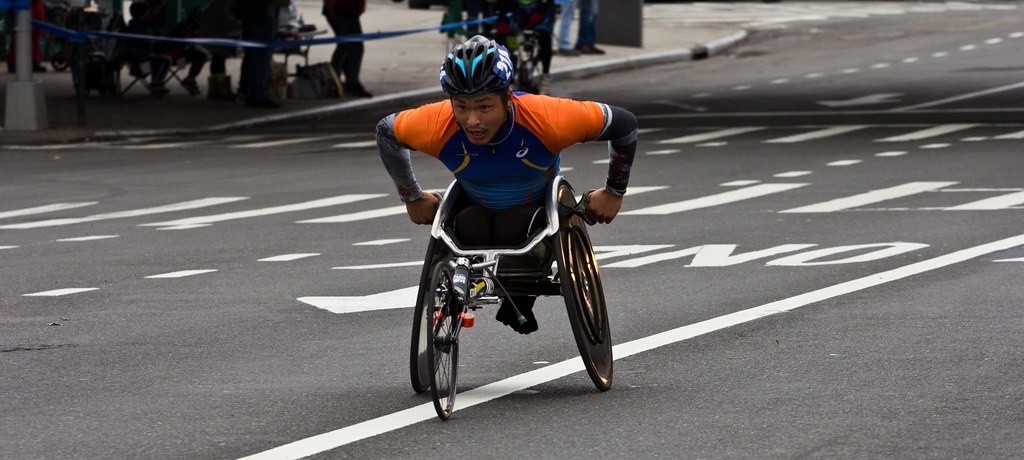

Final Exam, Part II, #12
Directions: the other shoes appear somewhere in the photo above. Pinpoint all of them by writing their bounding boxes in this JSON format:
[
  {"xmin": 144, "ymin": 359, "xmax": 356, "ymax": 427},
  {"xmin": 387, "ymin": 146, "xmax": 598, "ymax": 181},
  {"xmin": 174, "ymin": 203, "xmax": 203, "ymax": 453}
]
[
  {"xmin": 348, "ymin": 83, "xmax": 371, "ymax": 98},
  {"xmin": 560, "ymin": 49, "xmax": 577, "ymax": 56},
  {"xmin": 574, "ymin": 41, "xmax": 604, "ymax": 54},
  {"xmin": 242, "ymin": 96, "xmax": 281, "ymax": 109}
]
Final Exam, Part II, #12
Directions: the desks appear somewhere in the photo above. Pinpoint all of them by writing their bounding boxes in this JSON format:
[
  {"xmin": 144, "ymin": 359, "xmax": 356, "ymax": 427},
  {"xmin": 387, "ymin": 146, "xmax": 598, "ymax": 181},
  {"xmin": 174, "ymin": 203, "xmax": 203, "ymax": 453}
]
[{"xmin": 277, "ymin": 27, "xmax": 328, "ymax": 86}]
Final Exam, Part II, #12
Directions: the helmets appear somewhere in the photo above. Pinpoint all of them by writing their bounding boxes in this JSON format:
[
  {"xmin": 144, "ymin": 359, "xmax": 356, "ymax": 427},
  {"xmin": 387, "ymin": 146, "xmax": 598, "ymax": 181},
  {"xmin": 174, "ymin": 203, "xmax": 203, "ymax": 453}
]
[{"xmin": 439, "ymin": 34, "xmax": 514, "ymax": 96}]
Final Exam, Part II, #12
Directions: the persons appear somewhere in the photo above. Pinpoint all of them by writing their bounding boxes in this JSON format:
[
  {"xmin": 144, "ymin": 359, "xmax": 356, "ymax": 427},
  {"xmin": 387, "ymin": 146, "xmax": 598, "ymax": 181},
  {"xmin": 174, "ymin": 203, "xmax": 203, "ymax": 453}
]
[
  {"xmin": 8, "ymin": 0, "xmax": 607, "ymax": 109},
  {"xmin": 375, "ymin": 35, "xmax": 638, "ymax": 335}
]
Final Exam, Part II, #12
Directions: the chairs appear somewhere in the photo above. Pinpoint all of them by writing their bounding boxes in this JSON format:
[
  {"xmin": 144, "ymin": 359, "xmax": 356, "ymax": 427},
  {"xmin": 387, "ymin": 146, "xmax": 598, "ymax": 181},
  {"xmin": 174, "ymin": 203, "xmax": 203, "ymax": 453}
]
[{"xmin": 120, "ymin": 50, "xmax": 193, "ymax": 93}]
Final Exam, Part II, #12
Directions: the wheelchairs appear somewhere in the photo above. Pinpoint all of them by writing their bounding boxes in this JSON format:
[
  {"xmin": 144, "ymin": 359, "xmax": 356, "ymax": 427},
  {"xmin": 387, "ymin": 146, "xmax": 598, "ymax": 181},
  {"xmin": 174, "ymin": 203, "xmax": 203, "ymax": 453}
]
[{"xmin": 408, "ymin": 173, "xmax": 614, "ymax": 420}]
